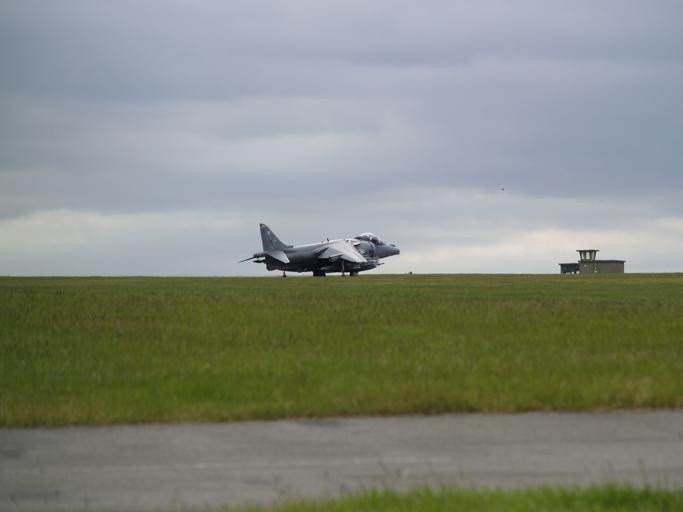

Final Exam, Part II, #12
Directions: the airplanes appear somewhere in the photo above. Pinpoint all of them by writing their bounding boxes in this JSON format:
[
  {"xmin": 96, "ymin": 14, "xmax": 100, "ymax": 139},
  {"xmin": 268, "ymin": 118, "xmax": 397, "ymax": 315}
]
[{"xmin": 235, "ymin": 219, "xmax": 402, "ymax": 275}]
